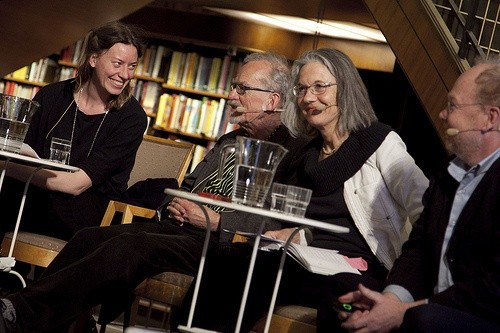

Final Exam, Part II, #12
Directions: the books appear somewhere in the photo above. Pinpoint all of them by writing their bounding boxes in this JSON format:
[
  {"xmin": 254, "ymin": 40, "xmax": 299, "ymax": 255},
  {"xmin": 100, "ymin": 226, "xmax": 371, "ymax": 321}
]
[
  {"xmin": 247, "ymin": 236, "xmax": 363, "ymax": 278},
  {"xmin": 0, "ymin": 39, "xmax": 241, "ymax": 173}
]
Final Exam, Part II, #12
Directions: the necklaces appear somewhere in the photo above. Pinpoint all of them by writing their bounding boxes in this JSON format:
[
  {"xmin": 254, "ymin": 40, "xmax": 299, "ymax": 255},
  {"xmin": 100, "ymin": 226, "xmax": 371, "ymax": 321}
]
[
  {"xmin": 68, "ymin": 86, "xmax": 110, "ymax": 166},
  {"xmin": 322, "ymin": 143, "xmax": 337, "ymax": 155}
]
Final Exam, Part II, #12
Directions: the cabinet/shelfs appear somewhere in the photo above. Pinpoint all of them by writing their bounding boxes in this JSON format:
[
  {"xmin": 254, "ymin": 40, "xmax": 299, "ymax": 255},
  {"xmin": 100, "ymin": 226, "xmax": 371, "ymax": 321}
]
[{"xmin": 3, "ymin": 28, "xmax": 229, "ymax": 177}]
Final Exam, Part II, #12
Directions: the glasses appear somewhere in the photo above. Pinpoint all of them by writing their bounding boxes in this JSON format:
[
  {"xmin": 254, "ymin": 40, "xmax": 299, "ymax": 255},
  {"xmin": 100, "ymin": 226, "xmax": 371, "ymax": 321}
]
[
  {"xmin": 230, "ymin": 82, "xmax": 275, "ymax": 95},
  {"xmin": 447, "ymin": 102, "xmax": 484, "ymax": 113},
  {"xmin": 292, "ymin": 82, "xmax": 337, "ymax": 97}
]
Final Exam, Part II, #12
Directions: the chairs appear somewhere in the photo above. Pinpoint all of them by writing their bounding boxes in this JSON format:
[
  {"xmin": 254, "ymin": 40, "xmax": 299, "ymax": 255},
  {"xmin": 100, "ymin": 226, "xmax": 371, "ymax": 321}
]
[
  {"xmin": 1, "ymin": 134, "xmax": 195, "ymax": 272},
  {"xmin": 233, "ymin": 229, "xmax": 317, "ymax": 333},
  {"xmin": 132, "ymin": 271, "xmax": 193, "ymax": 314}
]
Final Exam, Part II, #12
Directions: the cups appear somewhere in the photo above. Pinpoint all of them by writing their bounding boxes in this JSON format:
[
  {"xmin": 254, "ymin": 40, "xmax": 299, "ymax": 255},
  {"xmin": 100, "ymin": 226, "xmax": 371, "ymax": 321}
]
[
  {"xmin": 271, "ymin": 182, "xmax": 290, "ymax": 214},
  {"xmin": 285, "ymin": 185, "xmax": 313, "ymax": 218},
  {"xmin": 49, "ymin": 137, "xmax": 72, "ymax": 165}
]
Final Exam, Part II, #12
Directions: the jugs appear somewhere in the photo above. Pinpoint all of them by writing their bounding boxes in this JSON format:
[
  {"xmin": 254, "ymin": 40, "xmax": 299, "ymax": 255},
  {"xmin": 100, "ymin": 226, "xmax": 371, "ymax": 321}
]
[
  {"xmin": 0, "ymin": 92, "xmax": 40, "ymax": 153},
  {"xmin": 217, "ymin": 136, "xmax": 289, "ymax": 208}
]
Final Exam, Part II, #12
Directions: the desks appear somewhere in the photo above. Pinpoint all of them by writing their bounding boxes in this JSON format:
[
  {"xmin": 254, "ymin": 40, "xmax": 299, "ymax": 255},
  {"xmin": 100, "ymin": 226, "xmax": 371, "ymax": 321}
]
[
  {"xmin": 162, "ymin": 188, "xmax": 350, "ymax": 333},
  {"xmin": 0, "ymin": 150, "xmax": 79, "ymax": 291}
]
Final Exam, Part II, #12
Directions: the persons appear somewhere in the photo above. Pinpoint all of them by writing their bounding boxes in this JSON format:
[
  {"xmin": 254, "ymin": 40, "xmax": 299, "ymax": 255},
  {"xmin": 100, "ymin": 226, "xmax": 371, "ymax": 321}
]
[
  {"xmin": 0, "ymin": 50, "xmax": 317, "ymax": 333},
  {"xmin": 0, "ymin": 23, "xmax": 148, "ymax": 295},
  {"xmin": 168, "ymin": 48, "xmax": 431, "ymax": 333},
  {"xmin": 337, "ymin": 54, "xmax": 500, "ymax": 333}
]
